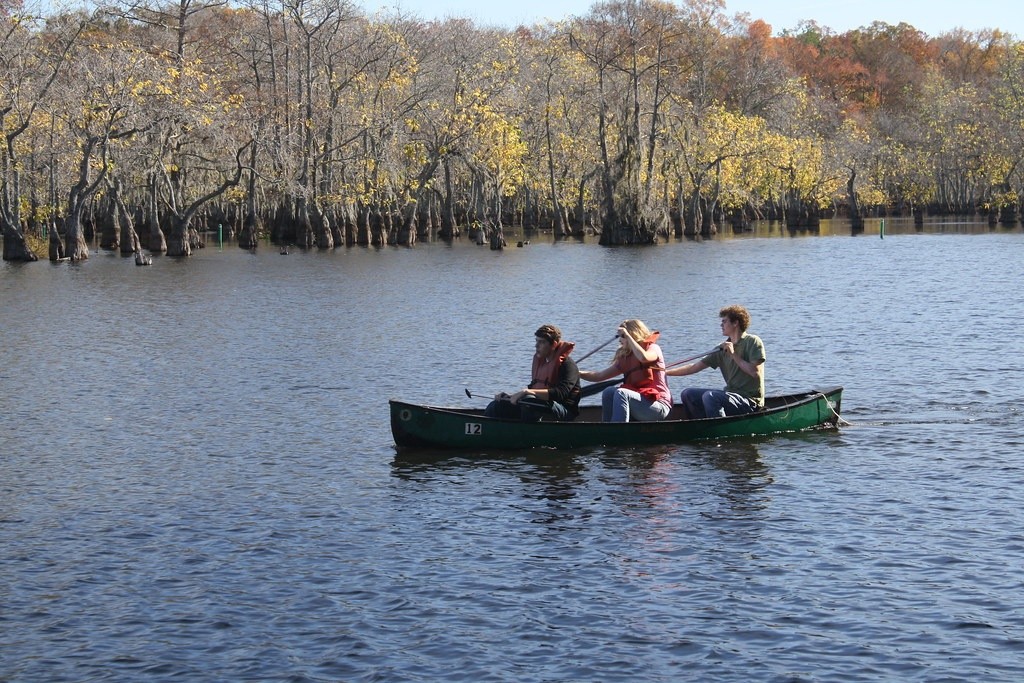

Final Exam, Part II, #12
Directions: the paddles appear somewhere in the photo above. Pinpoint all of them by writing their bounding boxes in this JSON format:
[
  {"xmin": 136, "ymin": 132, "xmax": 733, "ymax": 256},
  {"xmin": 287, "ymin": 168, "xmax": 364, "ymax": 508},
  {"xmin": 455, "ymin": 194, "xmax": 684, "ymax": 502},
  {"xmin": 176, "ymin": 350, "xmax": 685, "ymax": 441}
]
[
  {"xmin": 465, "ymin": 387, "xmax": 551, "ymax": 411},
  {"xmin": 574, "ymin": 331, "xmax": 623, "ymax": 365},
  {"xmin": 577, "ymin": 347, "xmax": 729, "ymax": 398}
]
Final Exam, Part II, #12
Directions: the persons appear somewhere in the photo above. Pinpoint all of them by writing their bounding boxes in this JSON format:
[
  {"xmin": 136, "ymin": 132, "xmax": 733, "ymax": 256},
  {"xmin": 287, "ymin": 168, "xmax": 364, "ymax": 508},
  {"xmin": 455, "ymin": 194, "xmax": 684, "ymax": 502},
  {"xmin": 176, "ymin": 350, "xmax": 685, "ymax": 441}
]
[
  {"xmin": 579, "ymin": 320, "xmax": 673, "ymax": 422},
  {"xmin": 666, "ymin": 305, "xmax": 765, "ymax": 418},
  {"xmin": 485, "ymin": 325, "xmax": 581, "ymax": 422}
]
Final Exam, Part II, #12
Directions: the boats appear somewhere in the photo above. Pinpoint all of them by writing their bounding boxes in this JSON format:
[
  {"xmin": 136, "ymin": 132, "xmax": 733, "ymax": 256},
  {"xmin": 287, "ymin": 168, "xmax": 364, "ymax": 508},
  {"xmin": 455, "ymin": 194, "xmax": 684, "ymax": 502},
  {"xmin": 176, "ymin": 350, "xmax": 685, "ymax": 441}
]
[{"xmin": 389, "ymin": 385, "xmax": 843, "ymax": 446}]
